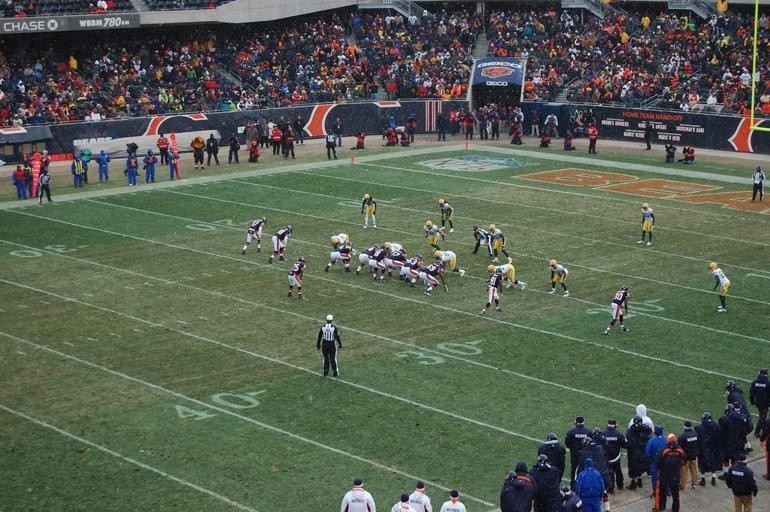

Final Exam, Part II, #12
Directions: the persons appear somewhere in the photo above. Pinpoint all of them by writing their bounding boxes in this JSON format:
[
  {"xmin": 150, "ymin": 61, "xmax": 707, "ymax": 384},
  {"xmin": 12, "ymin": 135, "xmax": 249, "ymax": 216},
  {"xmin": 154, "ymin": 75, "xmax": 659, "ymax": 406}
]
[
  {"xmin": 707, "ymin": 263, "xmax": 732, "ymax": 313},
  {"xmin": 241, "ymin": 207, "xmax": 528, "ymax": 317},
  {"xmin": 336, "ymin": 478, "xmax": 377, "ymax": 511},
  {"xmin": 392, "ymin": 495, "xmax": 418, "ymax": 512},
  {"xmin": 0, "ymin": 2, "xmax": 770, "ymax": 207},
  {"xmin": 547, "ymin": 260, "xmax": 570, "ymax": 297},
  {"xmin": 441, "ymin": 489, "xmax": 468, "ymax": 511},
  {"xmin": 599, "ymin": 286, "xmax": 633, "ymax": 335},
  {"xmin": 497, "ymin": 369, "xmax": 770, "ymax": 512},
  {"xmin": 407, "ymin": 479, "xmax": 433, "ymax": 511},
  {"xmin": 314, "ymin": 313, "xmax": 341, "ymax": 377},
  {"xmin": 636, "ymin": 199, "xmax": 657, "ymax": 249}
]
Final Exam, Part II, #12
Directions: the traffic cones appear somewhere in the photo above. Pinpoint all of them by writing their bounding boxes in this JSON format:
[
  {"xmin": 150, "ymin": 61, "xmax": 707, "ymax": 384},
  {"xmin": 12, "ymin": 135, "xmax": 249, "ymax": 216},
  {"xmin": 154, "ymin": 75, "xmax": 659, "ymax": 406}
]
[
  {"xmin": 465, "ymin": 141, "xmax": 470, "ymax": 149},
  {"xmin": 351, "ymin": 155, "xmax": 356, "ymax": 165}
]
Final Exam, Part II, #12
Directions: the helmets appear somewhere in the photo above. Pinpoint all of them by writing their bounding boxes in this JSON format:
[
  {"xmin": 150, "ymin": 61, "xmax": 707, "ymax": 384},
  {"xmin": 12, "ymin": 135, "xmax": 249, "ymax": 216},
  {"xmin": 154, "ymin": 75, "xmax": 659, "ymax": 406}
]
[
  {"xmin": 702, "ymin": 411, "xmax": 710, "ymax": 421},
  {"xmin": 329, "ymin": 235, "xmax": 446, "ymax": 267},
  {"xmin": 642, "ymin": 203, "xmax": 649, "ymax": 210},
  {"xmin": 364, "ymin": 193, "xmax": 370, "ymax": 200},
  {"xmin": 633, "ymin": 416, "xmax": 643, "ymax": 427},
  {"xmin": 550, "ymin": 259, "xmax": 557, "ymax": 267},
  {"xmin": 288, "ymin": 226, "xmax": 293, "ymax": 229},
  {"xmin": 262, "ymin": 217, "xmax": 267, "ymax": 223},
  {"xmin": 486, "ymin": 263, "xmax": 503, "ymax": 275},
  {"xmin": 536, "ymin": 433, "xmax": 559, "ymax": 468},
  {"xmin": 439, "ymin": 199, "xmax": 444, "ymax": 206},
  {"xmin": 723, "ymin": 380, "xmax": 743, "ymax": 414},
  {"xmin": 709, "ymin": 262, "xmax": 717, "ymax": 269},
  {"xmin": 622, "ymin": 286, "xmax": 628, "ymax": 291},
  {"xmin": 488, "ymin": 224, "xmax": 496, "ymax": 233},
  {"xmin": 426, "ymin": 221, "xmax": 432, "ymax": 228}
]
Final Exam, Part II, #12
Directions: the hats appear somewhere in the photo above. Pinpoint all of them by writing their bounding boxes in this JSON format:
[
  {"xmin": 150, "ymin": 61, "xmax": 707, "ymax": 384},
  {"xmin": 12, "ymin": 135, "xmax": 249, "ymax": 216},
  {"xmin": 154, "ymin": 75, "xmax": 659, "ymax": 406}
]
[
  {"xmin": 473, "ymin": 225, "xmax": 478, "ymax": 231},
  {"xmin": 516, "ymin": 460, "xmax": 527, "ymax": 471},
  {"xmin": 737, "ymin": 454, "xmax": 747, "ymax": 461},
  {"xmin": 353, "ymin": 477, "xmax": 364, "ymax": 488},
  {"xmin": 760, "ymin": 370, "xmax": 768, "ymax": 375},
  {"xmin": 400, "ymin": 481, "xmax": 459, "ymax": 503},
  {"xmin": 683, "ymin": 421, "xmax": 692, "ymax": 429},
  {"xmin": 575, "ymin": 416, "xmax": 585, "ymax": 424},
  {"xmin": 584, "ymin": 458, "xmax": 592, "ymax": 465},
  {"xmin": 326, "ymin": 315, "xmax": 333, "ymax": 321},
  {"xmin": 655, "ymin": 425, "xmax": 678, "ymax": 443},
  {"xmin": 607, "ymin": 418, "xmax": 617, "ymax": 427}
]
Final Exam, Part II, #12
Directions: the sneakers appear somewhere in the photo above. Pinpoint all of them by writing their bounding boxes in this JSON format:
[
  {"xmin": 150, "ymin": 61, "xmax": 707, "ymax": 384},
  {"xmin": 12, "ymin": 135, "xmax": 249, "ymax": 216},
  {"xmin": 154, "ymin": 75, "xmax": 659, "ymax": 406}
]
[
  {"xmin": 717, "ymin": 306, "xmax": 727, "ymax": 313},
  {"xmin": 440, "ymin": 226, "xmax": 454, "ymax": 233},
  {"xmin": 602, "ymin": 330, "xmax": 611, "ymax": 335},
  {"xmin": 647, "ymin": 243, "xmax": 653, "ymax": 246},
  {"xmin": 622, "ymin": 328, "xmax": 632, "ymax": 331},
  {"xmin": 636, "ymin": 240, "xmax": 645, "ymax": 244},
  {"xmin": 471, "ymin": 251, "xmax": 511, "ymax": 264},
  {"xmin": 506, "ymin": 282, "xmax": 527, "ymax": 290},
  {"xmin": 562, "ymin": 292, "xmax": 570, "ymax": 298},
  {"xmin": 288, "ymin": 291, "xmax": 303, "ymax": 299},
  {"xmin": 548, "ymin": 289, "xmax": 556, "ymax": 294},
  {"xmin": 363, "ymin": 224, "xmax": 377, "ymax": 229},
  {"xmin": 325, "ymin": 266, "xmax": 392, "ymax": 282},
  {"xmin": 480, "ymin": 284, "xmax": 504, "ymax": 316},
  {"xmin": 609, "ymin": 480, "xmax": 642, "ymax": 494},
  {"xmin": 459, "ymin": 271, "xmax": 465, "ymax": 276},
  {"xmin": 400, "ymin": 274, "xmax": 432, "ymax": 297},
  {"xmin": 692, "ymin": 473, "xmax": 725, "ymax": 489},
  {"xmin": 242, "ymin": 247, "xmax": 285, "ymax": 263}
]
